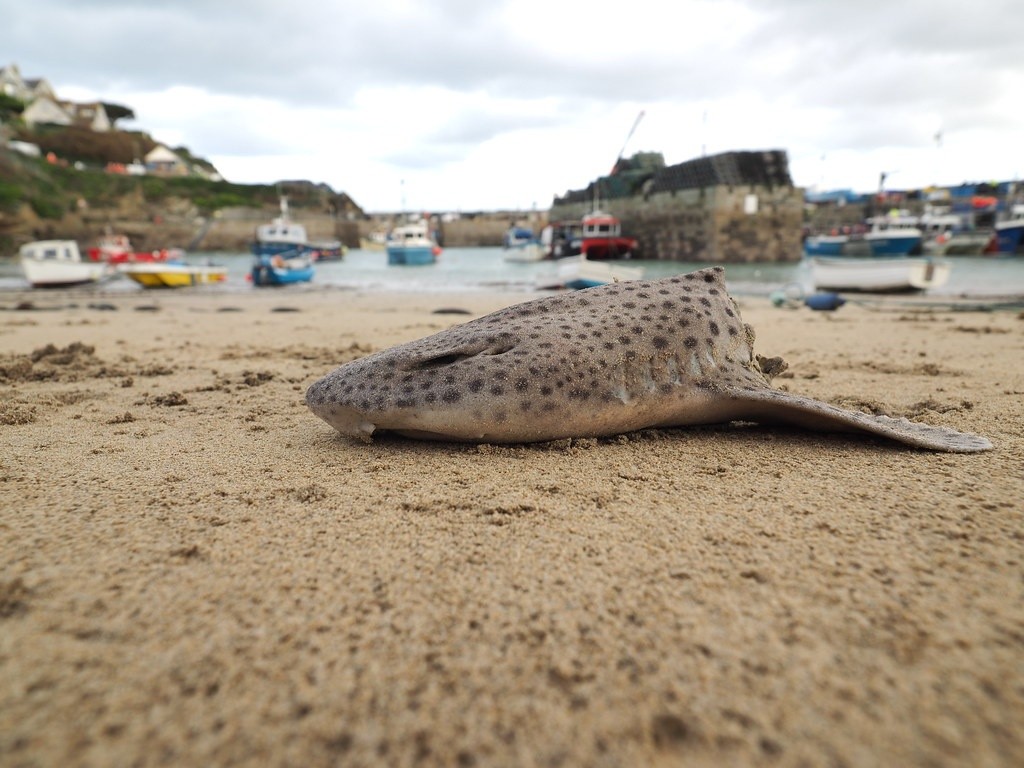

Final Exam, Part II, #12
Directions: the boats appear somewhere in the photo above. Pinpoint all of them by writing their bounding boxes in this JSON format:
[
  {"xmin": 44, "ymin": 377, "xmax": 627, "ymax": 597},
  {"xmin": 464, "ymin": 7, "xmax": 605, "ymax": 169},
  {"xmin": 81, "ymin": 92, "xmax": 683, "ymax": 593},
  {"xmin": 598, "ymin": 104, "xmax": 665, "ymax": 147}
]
[
  {"xmin": 386, "ymin": 213, "xmax": 440, "ymax": 265},
  {"xmin": 309, "ymin": 241, "xmax": 343, "ymax": 261},
  {"xmin": 118, "ymin": 263, "xmax": 228, "ymax": 287},
  {"xmin": 86, "ymin": 226, "xmax": 185, "ymax": 263},
  {"xmin": 504, "ymin": 209, "xmax": 644, "ymax": 292},
  {"xmin": 251, "ymin": 248, "xmax": 314, "ymax": 288},
  {"xmin": 17, "ymin": 238, "xmax": 117, "ymax": 284},
  {"xmin": 254, "ymin": 194, "xmax": 308, "ymax": 255}
]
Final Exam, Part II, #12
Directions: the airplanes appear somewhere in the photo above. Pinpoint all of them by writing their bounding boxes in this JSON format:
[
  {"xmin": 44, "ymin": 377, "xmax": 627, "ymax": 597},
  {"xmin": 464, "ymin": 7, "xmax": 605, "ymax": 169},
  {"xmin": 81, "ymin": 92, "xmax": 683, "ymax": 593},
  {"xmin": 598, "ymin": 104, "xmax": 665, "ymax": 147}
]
[{"xmin": 803, "ymin": 173, "xmax": 1024, "ymax": 293}]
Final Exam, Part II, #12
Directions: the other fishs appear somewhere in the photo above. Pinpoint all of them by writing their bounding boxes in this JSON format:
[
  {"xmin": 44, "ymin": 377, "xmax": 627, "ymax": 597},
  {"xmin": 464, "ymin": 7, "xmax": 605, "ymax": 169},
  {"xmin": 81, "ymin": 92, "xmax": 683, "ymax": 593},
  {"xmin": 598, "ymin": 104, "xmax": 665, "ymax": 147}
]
[{"xmin": 305, "ymin": 265, "xmax": 995, "ymax": 455}]
